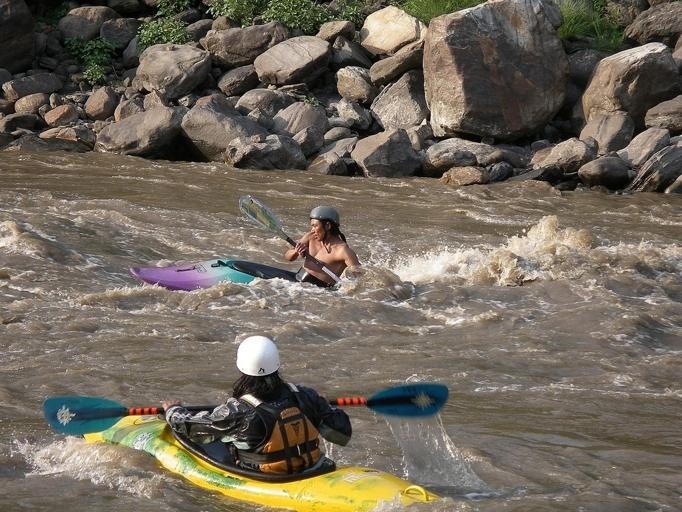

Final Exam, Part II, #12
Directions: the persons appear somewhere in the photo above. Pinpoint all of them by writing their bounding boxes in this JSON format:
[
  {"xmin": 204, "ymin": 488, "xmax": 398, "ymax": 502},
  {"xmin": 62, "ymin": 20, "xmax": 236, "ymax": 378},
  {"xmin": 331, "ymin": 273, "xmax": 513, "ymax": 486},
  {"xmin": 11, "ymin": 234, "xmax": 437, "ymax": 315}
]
[
  {"xmin": 159, "ymin": 336, "xmax": 352, "ymax": 474},
  {"xmin": 284, "ymin": 204, "xmax": 365, "ymax": 287}
]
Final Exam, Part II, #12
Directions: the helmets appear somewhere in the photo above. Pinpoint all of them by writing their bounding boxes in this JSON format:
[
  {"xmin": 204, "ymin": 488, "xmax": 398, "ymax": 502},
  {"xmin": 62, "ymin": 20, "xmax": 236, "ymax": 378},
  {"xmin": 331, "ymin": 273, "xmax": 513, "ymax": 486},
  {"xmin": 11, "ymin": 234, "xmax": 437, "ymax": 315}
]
[
  {"xmin": 310, "ymin": 206, "xmax": 340, "ymax": 227},
  {"xmin": 236, "ymin": 336, "xmax": 280, "ymax": 376}
]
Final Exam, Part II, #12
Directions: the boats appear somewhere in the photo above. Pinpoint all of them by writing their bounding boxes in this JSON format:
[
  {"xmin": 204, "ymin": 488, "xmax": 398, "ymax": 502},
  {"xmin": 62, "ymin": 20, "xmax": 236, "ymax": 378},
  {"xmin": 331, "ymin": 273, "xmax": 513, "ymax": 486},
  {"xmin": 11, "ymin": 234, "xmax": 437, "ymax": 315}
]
[
  {"xmin": 127, "ymin": 258, "xmax": 343, "ymax": 294},
  {"xmin": 78, "ymin": 409, "xmax": 447, "ymax": 512}
]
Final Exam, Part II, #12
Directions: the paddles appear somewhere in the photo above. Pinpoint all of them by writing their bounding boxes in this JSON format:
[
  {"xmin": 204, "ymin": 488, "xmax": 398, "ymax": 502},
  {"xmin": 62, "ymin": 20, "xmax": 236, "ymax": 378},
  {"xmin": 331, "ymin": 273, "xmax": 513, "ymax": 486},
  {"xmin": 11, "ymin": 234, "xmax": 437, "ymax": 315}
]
[
  {"xmin": 43, "ymin": 383, "xmax": 449, "ymax": 434},
  {"xmin": 238, "ymin": 193, "xmax": 342, "ymax": 283}
]
